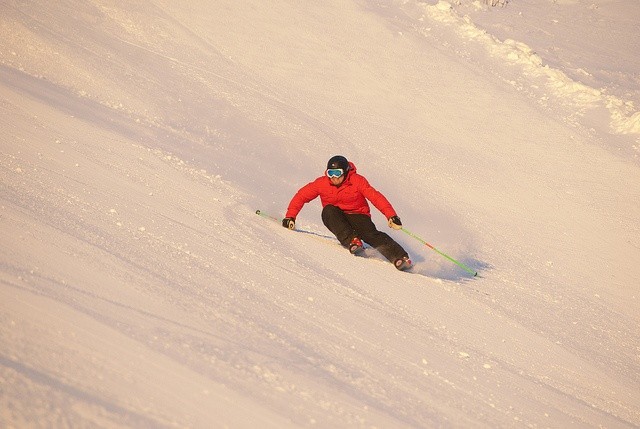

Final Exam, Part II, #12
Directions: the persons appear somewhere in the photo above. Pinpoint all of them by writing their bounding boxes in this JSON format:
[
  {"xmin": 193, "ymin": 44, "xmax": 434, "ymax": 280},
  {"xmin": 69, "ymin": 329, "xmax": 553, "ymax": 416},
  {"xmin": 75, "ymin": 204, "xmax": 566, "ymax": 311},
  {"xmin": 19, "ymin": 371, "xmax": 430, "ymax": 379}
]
[{"xmin": 286, "ymin": 154, "xmax": 413, "ymax": 271}]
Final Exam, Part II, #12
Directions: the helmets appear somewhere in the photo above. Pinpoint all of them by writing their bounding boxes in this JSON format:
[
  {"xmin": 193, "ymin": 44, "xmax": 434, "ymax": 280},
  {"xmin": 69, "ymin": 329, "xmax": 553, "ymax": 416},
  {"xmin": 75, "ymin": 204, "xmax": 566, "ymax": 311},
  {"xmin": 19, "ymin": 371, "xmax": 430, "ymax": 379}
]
[{"xmin": 327, "ymin": 155, "xmax": 348, "ymax": 175}]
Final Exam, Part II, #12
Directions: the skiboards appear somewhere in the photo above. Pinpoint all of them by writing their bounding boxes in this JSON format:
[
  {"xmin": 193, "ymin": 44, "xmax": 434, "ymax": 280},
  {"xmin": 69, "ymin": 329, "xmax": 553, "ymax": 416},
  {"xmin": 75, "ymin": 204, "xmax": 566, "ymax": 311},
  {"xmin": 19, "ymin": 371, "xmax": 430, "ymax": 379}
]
[{"xmin": 348, "ymin": 243, "xmax": 413, "ymax": 271}]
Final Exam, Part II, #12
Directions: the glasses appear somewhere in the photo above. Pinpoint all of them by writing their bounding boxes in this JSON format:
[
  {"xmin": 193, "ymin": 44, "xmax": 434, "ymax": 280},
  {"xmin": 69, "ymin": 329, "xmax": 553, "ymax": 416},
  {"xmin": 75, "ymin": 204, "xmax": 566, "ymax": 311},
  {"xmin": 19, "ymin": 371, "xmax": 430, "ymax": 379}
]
[{"xmin": 327, "ymin": 169, "xmax": 344, "ymax": 178}]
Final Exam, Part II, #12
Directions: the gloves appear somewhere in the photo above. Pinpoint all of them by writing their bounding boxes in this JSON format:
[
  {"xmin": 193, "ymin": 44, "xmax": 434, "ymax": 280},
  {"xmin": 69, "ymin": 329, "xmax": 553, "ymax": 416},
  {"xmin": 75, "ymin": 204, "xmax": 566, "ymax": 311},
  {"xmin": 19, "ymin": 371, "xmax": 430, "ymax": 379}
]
[
  {"xmin": 388, "ymin": 215, "xmax": 403, "ymax": 230},
  {"xmin": 282, "ymin": 217, "xmax": 295, "ymax": 230}
]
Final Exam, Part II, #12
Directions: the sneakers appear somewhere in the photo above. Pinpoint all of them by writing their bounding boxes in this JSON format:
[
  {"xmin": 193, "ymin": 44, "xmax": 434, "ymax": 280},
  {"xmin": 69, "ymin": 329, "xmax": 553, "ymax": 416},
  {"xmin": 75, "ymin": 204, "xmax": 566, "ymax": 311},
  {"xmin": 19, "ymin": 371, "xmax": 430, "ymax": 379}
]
[
  {"xmin": 395, "ymin": 257, "xmax": 413, "ymax": 271},
  {"xmin": 350, "ymin": 237, "xmax": 365, "ymax": 254}
]
